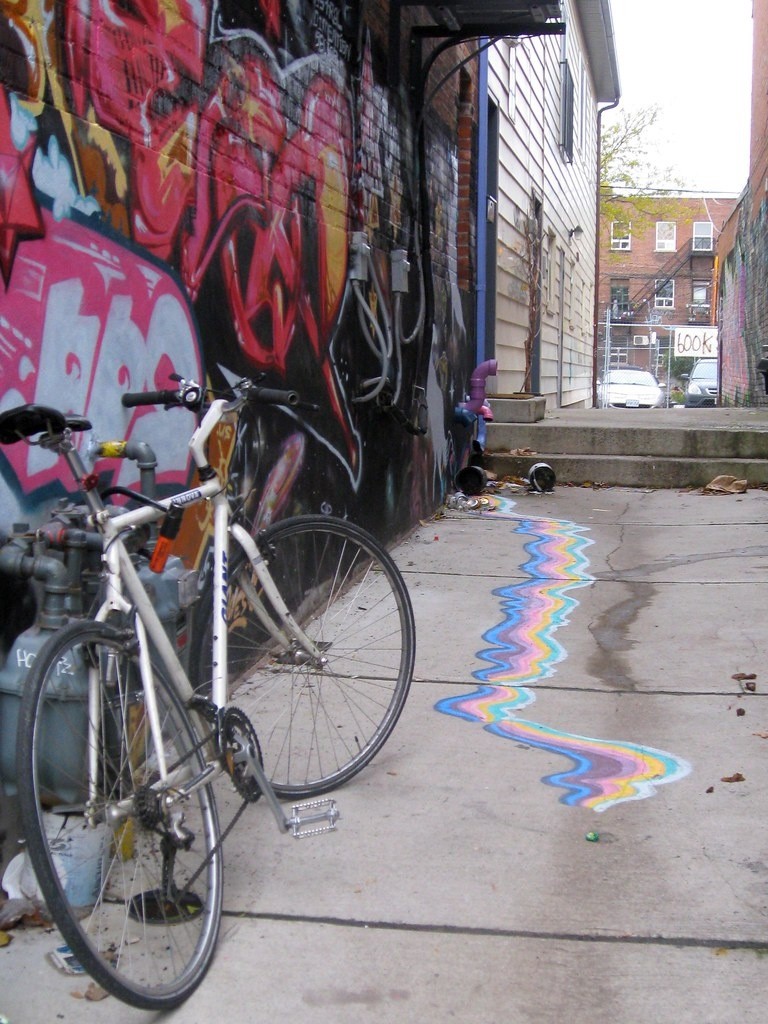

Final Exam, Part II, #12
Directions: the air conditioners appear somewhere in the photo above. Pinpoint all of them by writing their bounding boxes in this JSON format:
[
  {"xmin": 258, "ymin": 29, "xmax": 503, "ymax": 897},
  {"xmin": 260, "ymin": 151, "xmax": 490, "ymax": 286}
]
[
  {"xmin": 633, "ymin": 335, "xmax": 649, "ymax": 346},
  {"xmin": 658, "ymin": 240, "xmax": 675, "ymax": 250},
  {"xmin": 423, "ymin": 0, "xmax": 561, "ymax": 26}
]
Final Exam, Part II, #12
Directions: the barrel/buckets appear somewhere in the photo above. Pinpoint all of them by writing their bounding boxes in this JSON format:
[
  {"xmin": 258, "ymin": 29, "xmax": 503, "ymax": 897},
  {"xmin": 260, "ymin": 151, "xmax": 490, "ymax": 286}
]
[
  {"xmin": 528, "ymin": 462, "xmax": 555, "ymax": 492},
  {"xmin": 454, "ymin": 466, "xmax": 486, "ymax": 496}
]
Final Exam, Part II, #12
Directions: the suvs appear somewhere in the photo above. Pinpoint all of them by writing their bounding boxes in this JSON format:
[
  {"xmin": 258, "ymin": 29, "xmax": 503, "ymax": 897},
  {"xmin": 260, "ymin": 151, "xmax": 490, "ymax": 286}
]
[{"xmin": 680, "ymin": 359, "xmax": 718, "ymax": 408}]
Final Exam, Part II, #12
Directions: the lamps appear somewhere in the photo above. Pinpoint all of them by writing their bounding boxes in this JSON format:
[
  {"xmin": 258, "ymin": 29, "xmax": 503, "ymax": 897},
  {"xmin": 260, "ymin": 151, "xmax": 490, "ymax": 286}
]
[{"xmin": 569, "ymin": 225, "xmax": 583, "ymax": 240}]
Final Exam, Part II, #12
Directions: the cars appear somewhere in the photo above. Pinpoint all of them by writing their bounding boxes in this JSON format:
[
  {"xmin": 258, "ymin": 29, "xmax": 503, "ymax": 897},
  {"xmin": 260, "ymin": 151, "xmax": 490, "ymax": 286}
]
[{"xmin": 596, "ymin": 370, "xmax": 667, "ymax": 409}]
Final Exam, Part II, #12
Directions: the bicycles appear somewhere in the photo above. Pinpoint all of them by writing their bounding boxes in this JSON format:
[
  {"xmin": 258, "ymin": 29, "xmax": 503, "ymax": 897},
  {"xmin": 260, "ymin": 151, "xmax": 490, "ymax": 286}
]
[{"xmin": 1, "ymin": 373, "xmax": 418, "ymax": 1011}]
